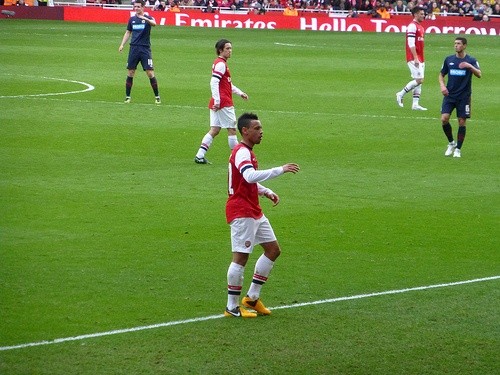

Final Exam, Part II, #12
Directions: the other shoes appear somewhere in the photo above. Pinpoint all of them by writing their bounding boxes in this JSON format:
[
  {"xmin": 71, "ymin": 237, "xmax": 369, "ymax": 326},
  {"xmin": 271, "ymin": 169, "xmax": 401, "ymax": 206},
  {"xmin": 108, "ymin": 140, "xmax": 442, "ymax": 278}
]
[
  {"xmin": 124, "ymin": 96, "xmax": 131, "ymax": 103},
  {"xmin": 445, "ymin": 140, "xmax": 457, "ymax": 156},
  {"xmin": 195, "ymin": 157, "xmax": 212, "ymax": 165},
  {"xmin": 155, "ymin": 96, "xmax": 161, "ymax": 104},
  {"xmin": 412, "ymin": 104, "xmax": 427, "ymax": 111},
  {"xmin": 453, "ymin": 148, "xmax": 461, "ymax": 157},
  {"xmin": 396, "ymin": 92, "xmax": 404, "ymax": 107}
]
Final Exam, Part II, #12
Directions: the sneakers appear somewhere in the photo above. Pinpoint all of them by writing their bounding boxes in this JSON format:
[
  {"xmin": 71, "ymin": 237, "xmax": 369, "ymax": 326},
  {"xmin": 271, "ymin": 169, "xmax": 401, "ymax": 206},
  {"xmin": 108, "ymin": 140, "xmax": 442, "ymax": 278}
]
[
  {"xmin": 224, "ymin": 306, "xmax": 257, "ymax": 318},
  {"xmin": 242, "ymin": 294, "xmax": 271, "ymax": 315}
]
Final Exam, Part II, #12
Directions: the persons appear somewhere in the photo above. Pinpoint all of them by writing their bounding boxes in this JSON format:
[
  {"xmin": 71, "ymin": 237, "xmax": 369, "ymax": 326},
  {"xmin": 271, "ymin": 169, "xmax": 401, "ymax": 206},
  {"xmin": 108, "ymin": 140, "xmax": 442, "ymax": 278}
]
[
  {"xmin": 194, "ymin": 39, "xmax": 248, "ymax": 164},
  {"xmin": 439, "ymin": 37, "xmax": 481, "ymax": 157},
  {"xmin": 119, "ymin": 0, "xmax": 161, "ymax": 104},
  {"xmin": 225, "ymin": 114, "xmax": 300, "ymax": 317},
  {"xmin": 395, "ymin": 6, "xmax": 428, "ymax": 110},
  {"xmin": 16, "ymin": 0, "xmax": 28, "ymax": 7},
  {"xmin": 143, "ymin": 0, "xmax": 500, "ymax": 21}
]
